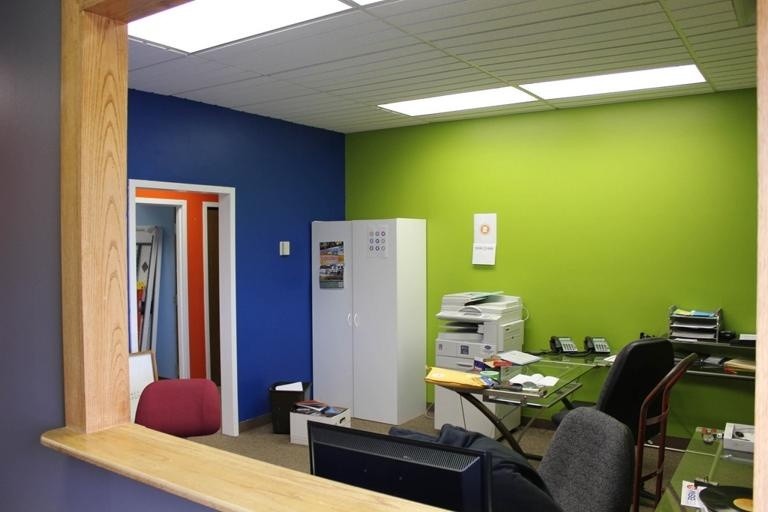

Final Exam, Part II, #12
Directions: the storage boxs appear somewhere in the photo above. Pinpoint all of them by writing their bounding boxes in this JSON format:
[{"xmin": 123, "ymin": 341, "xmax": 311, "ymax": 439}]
[{"xmin": 290, "ymin": 406, "xmax": 351, "ymax": 446}]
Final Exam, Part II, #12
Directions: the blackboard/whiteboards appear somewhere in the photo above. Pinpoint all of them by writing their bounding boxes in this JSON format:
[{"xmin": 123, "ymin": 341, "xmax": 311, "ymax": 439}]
[
  {"xmin": 584, "ymin": 336, "xmax": 610, "ymax": 354},
  {"xmin": 128, "ymin": 351, "xmax": 159, "ymax": 423}
]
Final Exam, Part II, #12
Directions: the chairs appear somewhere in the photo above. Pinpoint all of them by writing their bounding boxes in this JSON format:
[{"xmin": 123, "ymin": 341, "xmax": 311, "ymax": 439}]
[
  {"xmin": 134, "ymin": 376, "xmax": 224, "ymax": 437},
  {"xmin": 535, "ymin": 405, "xmax": 637, "ymax": 512},
  {"xmin": 550, "ymin": 335, "xmax": 676, "ymax": 511}
]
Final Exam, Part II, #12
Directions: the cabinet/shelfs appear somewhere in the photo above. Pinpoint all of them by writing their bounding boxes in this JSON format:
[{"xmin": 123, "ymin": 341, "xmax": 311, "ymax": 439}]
[{"xmin": 311, "ymin": 218, "xmax": 427, "ymax": 426}]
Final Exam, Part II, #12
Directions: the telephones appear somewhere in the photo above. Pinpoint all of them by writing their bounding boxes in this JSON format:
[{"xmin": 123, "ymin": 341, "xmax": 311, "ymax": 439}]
[{"xmin": 549, "ymin": 336, "xmax": 578, "ymax": 353}]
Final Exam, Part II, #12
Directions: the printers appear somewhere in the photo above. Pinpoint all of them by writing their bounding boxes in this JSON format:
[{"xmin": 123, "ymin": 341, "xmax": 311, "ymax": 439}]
[{"xmin": 433, "ymin": 290, "xmax": 525, "ymax": 441}]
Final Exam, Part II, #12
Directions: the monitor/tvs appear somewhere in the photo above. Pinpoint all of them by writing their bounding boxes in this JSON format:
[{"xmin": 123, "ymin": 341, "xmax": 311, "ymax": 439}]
[{"xmin": 306, "ymin": 418, "xmax": 495, "ymax": 512}]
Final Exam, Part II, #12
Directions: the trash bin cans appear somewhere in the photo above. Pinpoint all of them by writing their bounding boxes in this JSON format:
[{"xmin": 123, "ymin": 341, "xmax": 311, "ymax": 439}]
[{"xmin": 268, "ymin": 381, "xmax": 312, "ymax": 434}]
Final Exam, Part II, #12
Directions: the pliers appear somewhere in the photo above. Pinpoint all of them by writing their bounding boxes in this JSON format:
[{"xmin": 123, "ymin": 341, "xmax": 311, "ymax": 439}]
[{"xmin": 701, "ymin": 423, "xmax": 717, "ymax": 444}]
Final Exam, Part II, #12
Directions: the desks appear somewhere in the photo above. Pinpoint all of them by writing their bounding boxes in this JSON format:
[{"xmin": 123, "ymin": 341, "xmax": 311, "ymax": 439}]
[
  {"xmin": 652, "ymin": 424, "xmax": 754, "ymax": 511},
  {"xmin": 427, "ymin": 348, "xmax": 752, "ymax": 457}
]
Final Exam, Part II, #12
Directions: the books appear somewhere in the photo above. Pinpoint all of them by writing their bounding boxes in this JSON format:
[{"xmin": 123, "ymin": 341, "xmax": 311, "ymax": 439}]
[
  {"xmin": 496, "ymin": 349, "xmax": 541, "ymax": 367},
  {"xmin": 295, "ymin": 398, "xmax": 328, "ymax": 412},
  {"xmin": 725, "ymin": 358, "xmax": 755, "ymax": 371}
]
